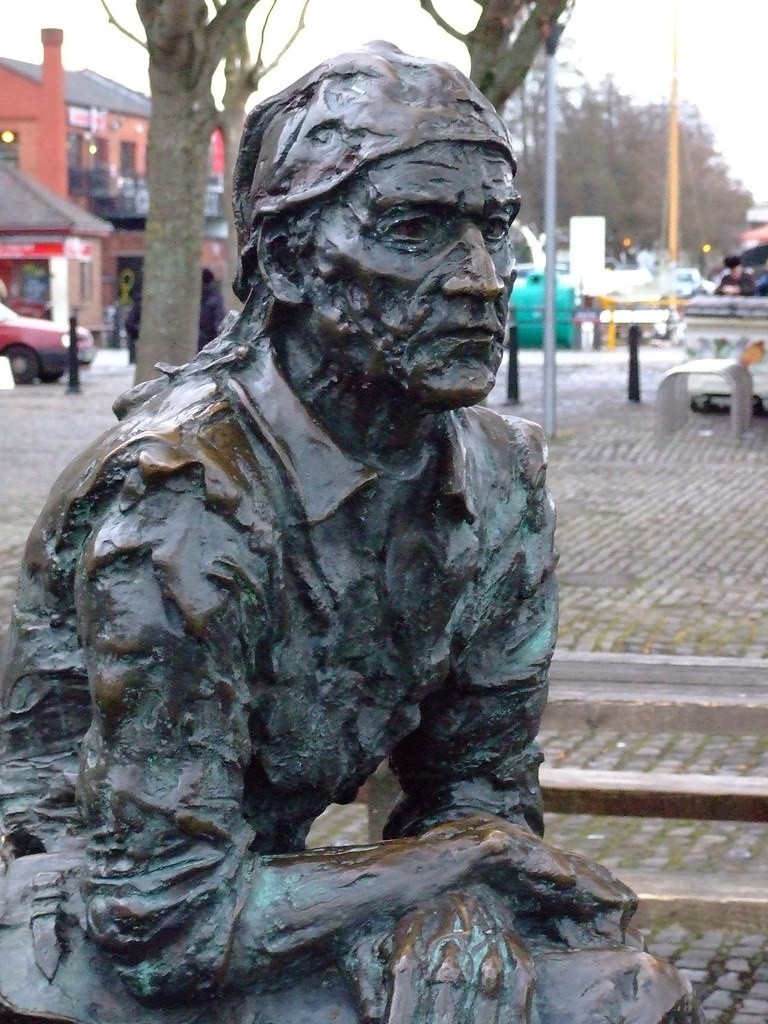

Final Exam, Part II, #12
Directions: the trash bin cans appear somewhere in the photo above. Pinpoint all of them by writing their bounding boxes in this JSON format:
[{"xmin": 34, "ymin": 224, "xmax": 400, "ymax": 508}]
[{"xmin": 581, "ymin": 320, "xmax": 595, "ymax": 352}]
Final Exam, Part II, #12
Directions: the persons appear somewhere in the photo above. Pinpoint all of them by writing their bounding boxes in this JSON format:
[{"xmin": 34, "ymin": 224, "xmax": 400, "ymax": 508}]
[
  {"xmin": 715, "ymin": 256, "xmax": 757, "ymax": 295},
  {"xmin": 0, "ymin": 39, "xmax": 708, "ymax": 1024},
  {"xmin": 198, "ymin": 265, "xmax": 224, "ymax": 354}
]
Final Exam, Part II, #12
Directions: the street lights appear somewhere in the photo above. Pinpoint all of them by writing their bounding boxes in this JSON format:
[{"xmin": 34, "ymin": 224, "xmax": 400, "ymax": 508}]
[{"xmin": 530, "ymin": 0, "xmax": 574, "ymax": 439}]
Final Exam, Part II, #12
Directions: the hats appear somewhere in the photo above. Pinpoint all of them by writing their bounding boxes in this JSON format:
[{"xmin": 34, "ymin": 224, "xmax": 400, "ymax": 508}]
[{"xmin": 725, "ymin": 256, "xmax": 740, "ymax": 266}]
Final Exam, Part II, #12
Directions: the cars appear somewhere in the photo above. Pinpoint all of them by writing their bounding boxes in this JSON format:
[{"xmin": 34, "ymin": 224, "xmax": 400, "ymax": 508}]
[
  {"xmin": 511, "ymin": 227, "xmax": 702, "ymax": 299},
  {"xmin": 0, "ymin": 302, "xmax": 96, "ymax": 386}
]
